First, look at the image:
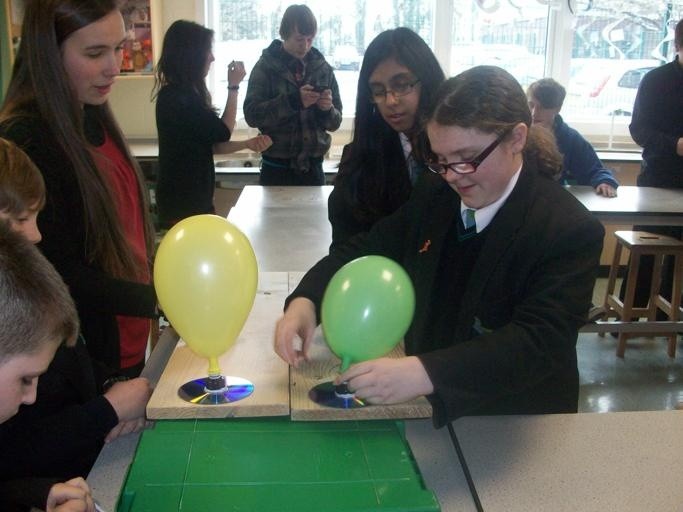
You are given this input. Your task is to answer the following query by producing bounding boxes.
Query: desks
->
[124,139,644,189]
[445,326,683,511]
[235,184,683,229]
[85,206,480,512]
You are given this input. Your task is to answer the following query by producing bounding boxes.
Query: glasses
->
[422,129,510,175]
[366,78,421,104]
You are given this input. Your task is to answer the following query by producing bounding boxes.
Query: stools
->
[597,230,683,358]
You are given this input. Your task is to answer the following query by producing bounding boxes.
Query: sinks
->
[593,150,642,155]
[213,156,262,175]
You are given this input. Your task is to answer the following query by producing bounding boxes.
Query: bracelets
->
[226,85,240,89]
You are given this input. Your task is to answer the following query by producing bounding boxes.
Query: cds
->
[178,375,253,405]
[308,381,368,409]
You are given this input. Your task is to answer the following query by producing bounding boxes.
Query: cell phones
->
[315,84,329,93]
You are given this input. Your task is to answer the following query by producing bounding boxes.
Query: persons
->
[1,220,96,512]
[273,64,608,412]
[2,4,164,384]
[526,77,618,325]
[0,134,155,511]
[149,20,272,230]
[243,3,343,186]
[329,27,458,256]
[619,19,683,333]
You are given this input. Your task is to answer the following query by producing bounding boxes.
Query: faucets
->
[609,112,618,150]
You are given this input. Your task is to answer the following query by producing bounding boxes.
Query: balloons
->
[153,214,259,378]
[320,255,417,372]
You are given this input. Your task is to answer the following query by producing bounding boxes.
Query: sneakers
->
[588,300,607,322]
